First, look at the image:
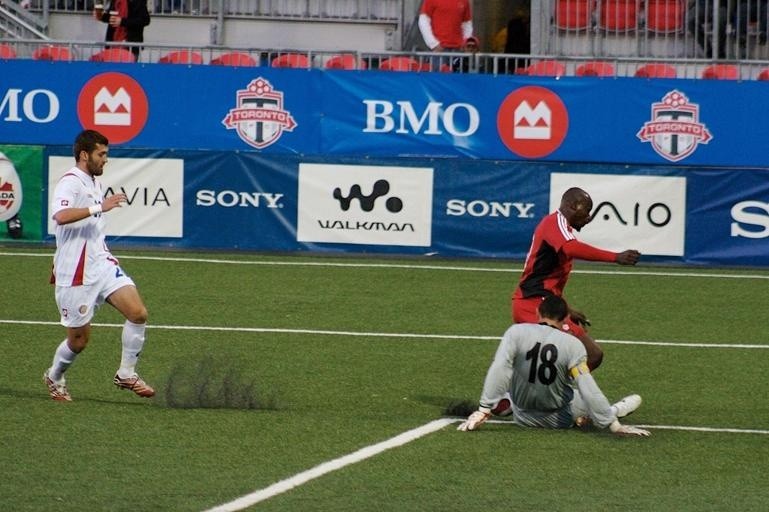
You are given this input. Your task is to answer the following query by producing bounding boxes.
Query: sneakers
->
[612,394,642,418]
[43,368,73,401]
[113,370,155,398]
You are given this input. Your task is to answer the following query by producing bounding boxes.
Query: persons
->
[456,294,650,437]
[418,0,484,73]
[94,0,149,56]
[490,188,641,417]
[42,129,156,403]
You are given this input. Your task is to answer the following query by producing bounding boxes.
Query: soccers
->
[490,388,514,416]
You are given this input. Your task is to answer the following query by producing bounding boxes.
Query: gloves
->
[456,405,491,432]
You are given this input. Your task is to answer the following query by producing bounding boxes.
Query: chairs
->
[0,0,769,80]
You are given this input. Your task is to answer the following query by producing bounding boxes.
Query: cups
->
[108,11,116,17]
[93,6,105,18]
